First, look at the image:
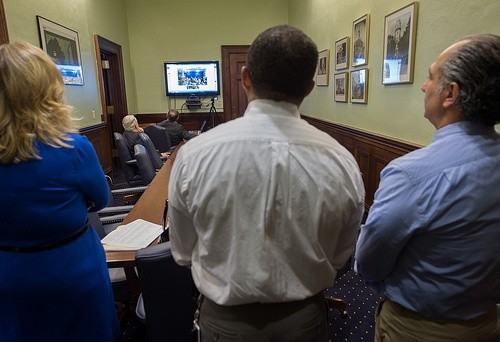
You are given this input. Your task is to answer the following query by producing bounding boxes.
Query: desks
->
[99,131,208,266]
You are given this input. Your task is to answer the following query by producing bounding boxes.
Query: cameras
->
[211,98,218,101]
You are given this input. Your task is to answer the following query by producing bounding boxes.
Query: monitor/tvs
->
[164,61,220,96]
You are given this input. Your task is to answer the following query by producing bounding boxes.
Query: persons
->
[167,25,366,342]
[122,114,169,174]
[354,30,364,59]
[354,33,500,342]
[0,40,121,342]
[158,109,201,147]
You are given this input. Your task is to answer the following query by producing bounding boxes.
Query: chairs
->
[104,172,148,216]
[87,211,118,241]
[139,131,165,172]
[144,124,176,154]
[133,144,158,184]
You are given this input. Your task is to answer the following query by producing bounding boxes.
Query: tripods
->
[200,101,220,132]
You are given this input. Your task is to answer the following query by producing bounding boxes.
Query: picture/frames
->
[349,67,369,104]
[381,1,420,86]
[333,71,348,103]
[316,49,331,87]
[35,15,85,87]
[350,12,370,67]
[334,36,350,70]
